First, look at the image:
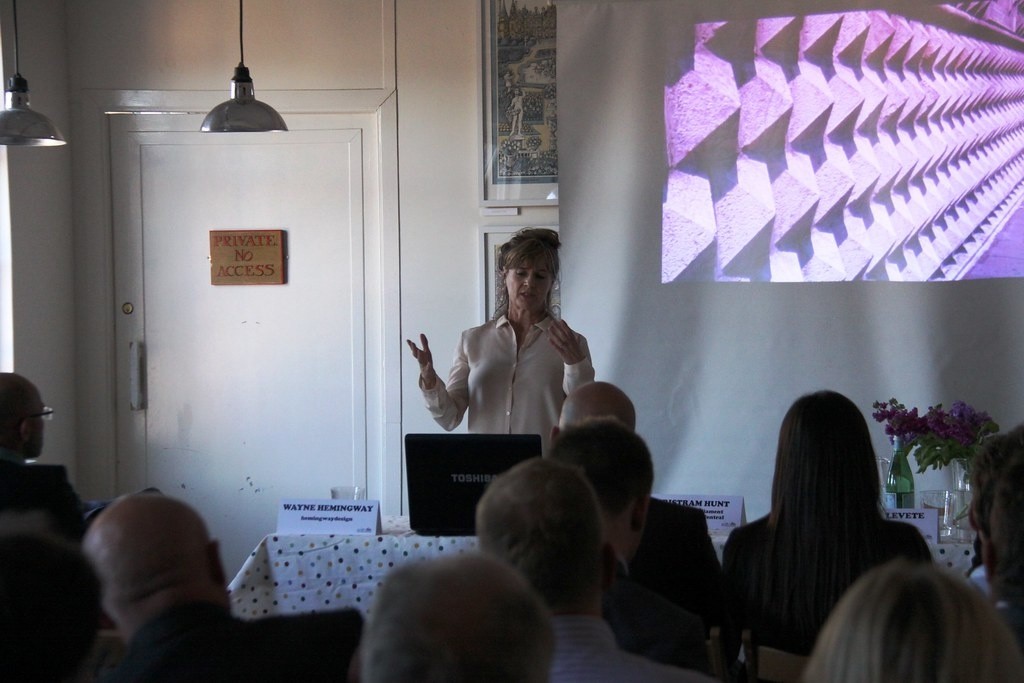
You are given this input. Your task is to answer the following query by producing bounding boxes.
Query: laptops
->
[404,433,542,537]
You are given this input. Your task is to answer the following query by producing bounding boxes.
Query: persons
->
[724,392,936,683]
[798,556,1024,683]
[468,455,723,683]
[0,512,107,683]
[405,227,596,468]
[351,549,556,683]
[546,381,728,643]
[83,490,365,682]
[963,424,1024,625]
[0,373,167,545]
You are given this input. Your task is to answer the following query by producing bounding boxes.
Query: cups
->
[875,457,888,496]
[921,491,977,544]
[331,487,365,501]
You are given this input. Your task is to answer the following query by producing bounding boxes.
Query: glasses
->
[14,406,52,428]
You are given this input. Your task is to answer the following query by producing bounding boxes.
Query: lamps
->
[1,0,67,145]
[198,0,289,132]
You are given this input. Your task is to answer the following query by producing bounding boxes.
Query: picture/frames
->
[941,458,977,543]
[473,0,559,206]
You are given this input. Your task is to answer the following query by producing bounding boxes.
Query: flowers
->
[872,398,942,455]
[914,401,1000,474]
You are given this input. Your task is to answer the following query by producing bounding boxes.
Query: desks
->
[228,514,976,620]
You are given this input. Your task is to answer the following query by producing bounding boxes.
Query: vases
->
[886,436,914,508]
